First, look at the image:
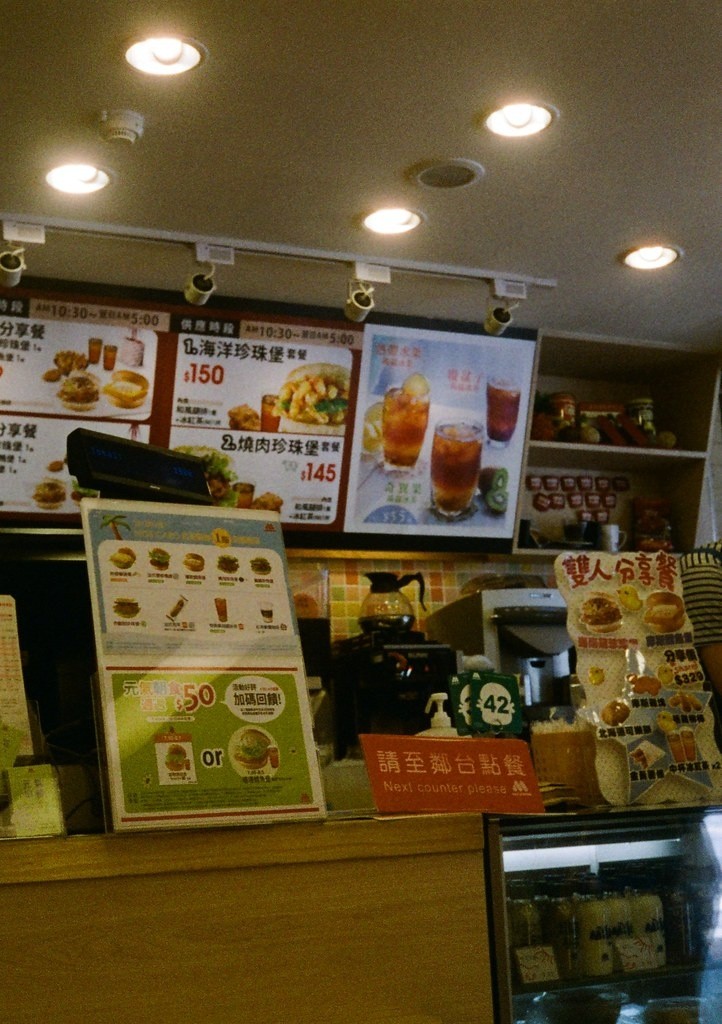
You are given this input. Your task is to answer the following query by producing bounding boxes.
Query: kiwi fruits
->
[478,466,510,514]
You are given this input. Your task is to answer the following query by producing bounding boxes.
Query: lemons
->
[402,372,430,395]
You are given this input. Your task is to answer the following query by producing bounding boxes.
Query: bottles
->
[507,881,702,994]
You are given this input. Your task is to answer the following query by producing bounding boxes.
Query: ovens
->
[422,585,585,729]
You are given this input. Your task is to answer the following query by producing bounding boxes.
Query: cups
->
[681,731,697,762]
[214,598,228,622]
[485,378,522,449]
[261,393,281,434]
[102,344,117,370]
[88,339,103,363]
[601,523,628,554]
[185,758,191,771]
[268,747,279,768]
[259,601,274,623]
[668,733,686,763]
[430,417,484,518]
[383,386,431,472]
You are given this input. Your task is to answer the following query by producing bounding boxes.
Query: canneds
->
[544,391,656,438]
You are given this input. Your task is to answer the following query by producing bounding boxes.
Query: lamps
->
[0,241,28,288]
[484,298,520,337]
[344,279,375,323]
[183,263,216,307]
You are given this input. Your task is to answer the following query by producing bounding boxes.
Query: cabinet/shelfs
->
[511,326,722,556]
[486,804,722,1024]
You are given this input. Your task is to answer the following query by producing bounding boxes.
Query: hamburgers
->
[102,370,149,409]
[270,362,352,436]
[31,478,67,510]
[234,729,271,770]
[578,592,686,634]
[147,548,272,576]
[164,743,187,772]
[112,598,141,618]
[70,480,99,507]
[55,370,100,410]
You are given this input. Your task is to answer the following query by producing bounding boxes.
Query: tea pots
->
[358,570,427,636]
